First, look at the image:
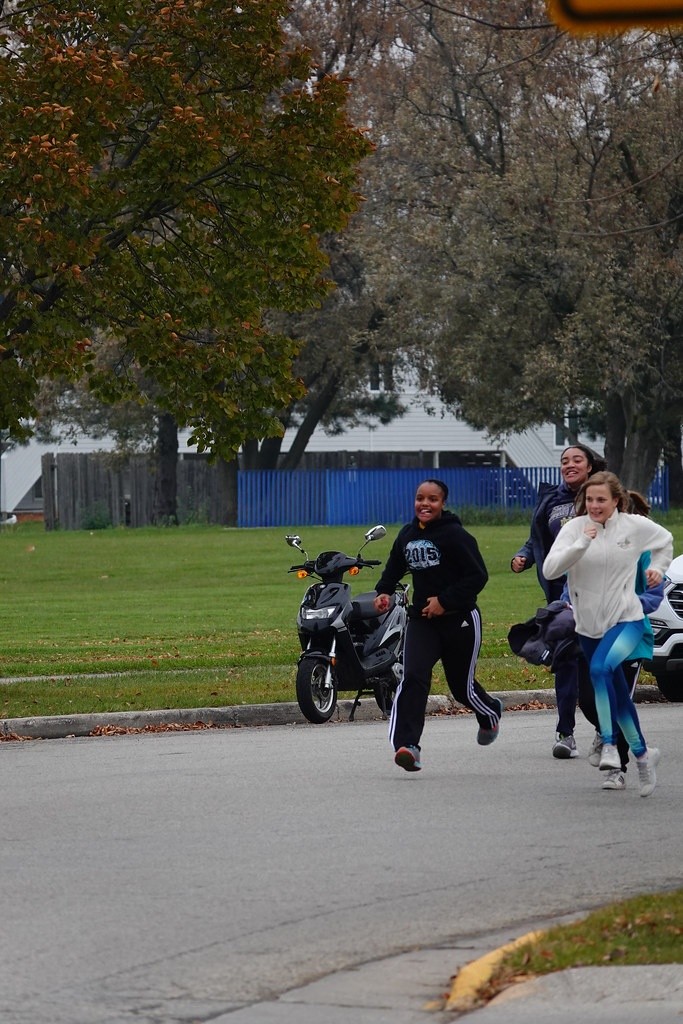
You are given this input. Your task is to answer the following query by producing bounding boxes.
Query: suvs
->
[638,555,683,703]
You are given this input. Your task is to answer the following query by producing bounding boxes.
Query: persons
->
[375,479,502,771]
[511,446,607,758]
[542,470,674,796]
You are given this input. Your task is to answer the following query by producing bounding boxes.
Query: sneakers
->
[588,731,603,767]
[553,735,579,759]
[602,768,627,789]
[598,743,621,771]
[477,698,502,746]
[395,747,422,773]
[636,747,660,796]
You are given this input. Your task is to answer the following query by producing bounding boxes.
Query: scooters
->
[284,524,411,725]
